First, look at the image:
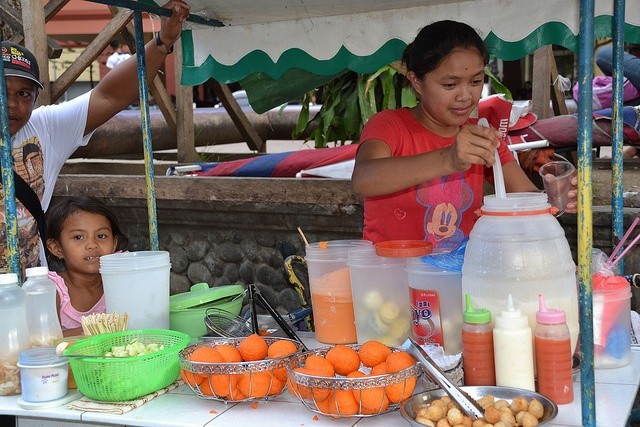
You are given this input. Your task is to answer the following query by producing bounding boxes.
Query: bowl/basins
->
[170,287,246,338]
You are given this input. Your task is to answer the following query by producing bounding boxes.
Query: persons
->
[45,194,129,337]
[0,1,191,276]
[106,39,134,109]
[350,19,578,252]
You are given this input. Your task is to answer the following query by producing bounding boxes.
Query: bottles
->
[461,292,495,387]
[0,273,29,397]
[534,293,574,405]
[492,293,535,392]
[25,265,63,356]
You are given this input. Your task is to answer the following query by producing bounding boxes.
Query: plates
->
[398,385,559,427]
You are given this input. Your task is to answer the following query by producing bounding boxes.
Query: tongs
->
[247,282,310,353]
[398,336,491,423]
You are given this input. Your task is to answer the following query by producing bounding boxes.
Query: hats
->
[0,40,45,91]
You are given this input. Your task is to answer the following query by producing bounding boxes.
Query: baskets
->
[63,329,191,401]
[286,341,422,418]
[178,335,304,403]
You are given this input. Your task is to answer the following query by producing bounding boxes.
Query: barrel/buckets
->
[462,190,579,360]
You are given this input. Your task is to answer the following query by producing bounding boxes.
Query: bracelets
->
[155,31,174,55]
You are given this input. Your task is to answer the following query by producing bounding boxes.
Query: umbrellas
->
[295,91,550,180]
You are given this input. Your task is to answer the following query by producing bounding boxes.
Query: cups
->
[587,269,634,370]
[15,344,71,404]
[538,160,575,211]
